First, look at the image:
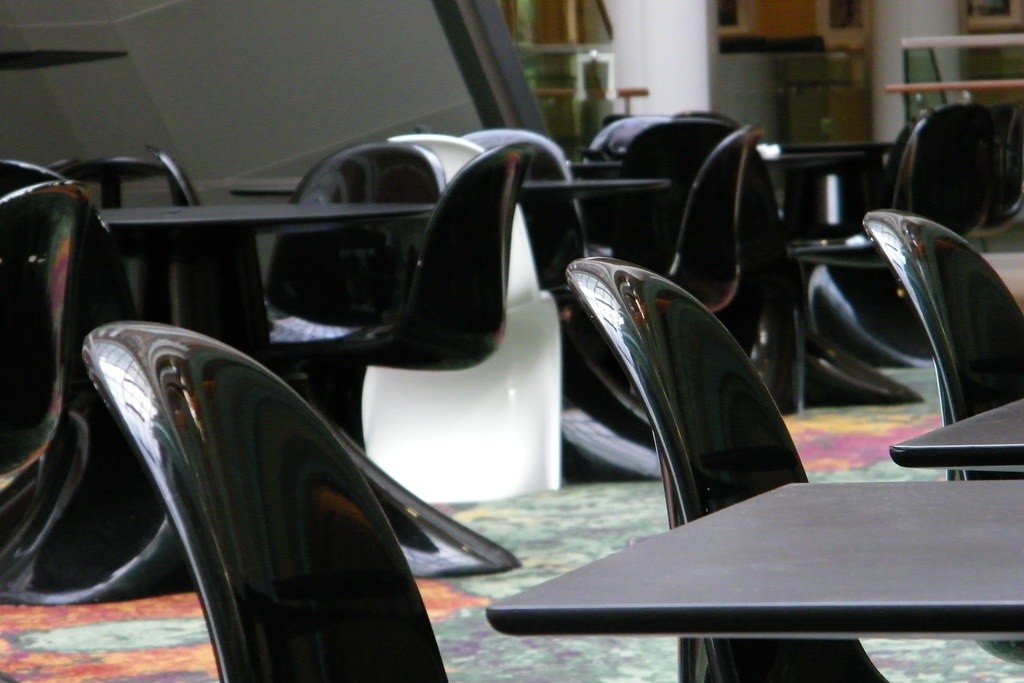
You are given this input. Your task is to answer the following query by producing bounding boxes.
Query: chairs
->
[0,102,1024,683]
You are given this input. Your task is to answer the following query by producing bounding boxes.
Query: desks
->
[890,398,1024,471]
[67,203,521,606]
[566,152,923,407]
[486,482,1024,683]
[228,179,667,477]
[757,142,929,367]
